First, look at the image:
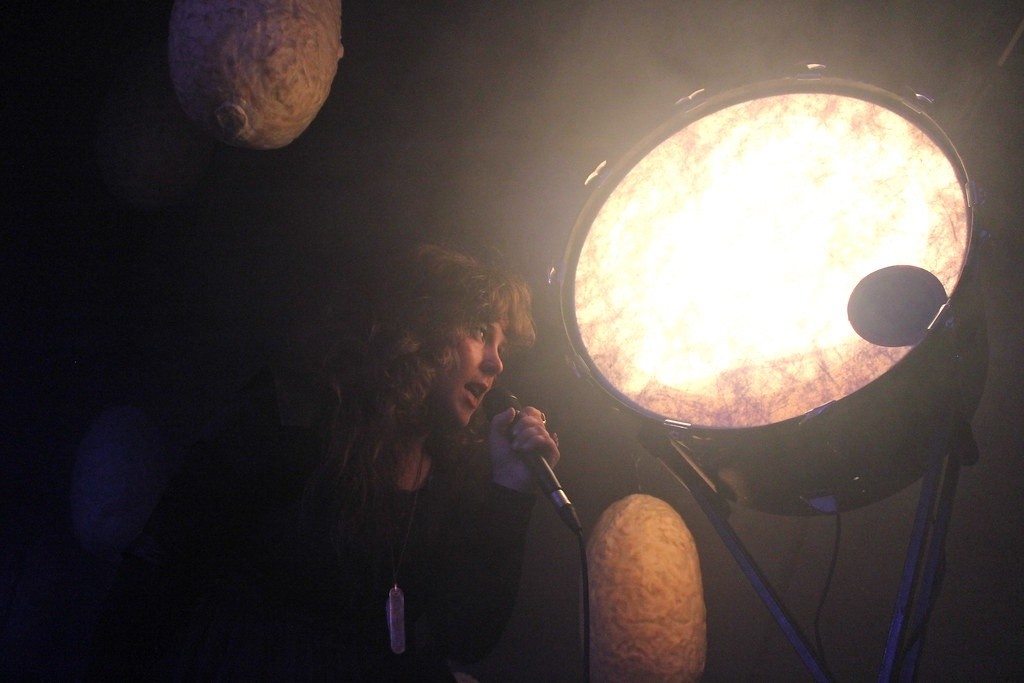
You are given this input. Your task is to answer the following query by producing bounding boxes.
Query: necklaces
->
[362,439,429,656]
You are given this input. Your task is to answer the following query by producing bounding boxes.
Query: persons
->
[83,242,562,683]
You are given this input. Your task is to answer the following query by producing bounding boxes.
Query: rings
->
[541,412,547,426]
[552,432,559,447]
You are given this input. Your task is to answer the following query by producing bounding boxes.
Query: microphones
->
[483,385,581,534]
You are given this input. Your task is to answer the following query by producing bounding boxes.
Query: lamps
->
[549,62,985,683]
